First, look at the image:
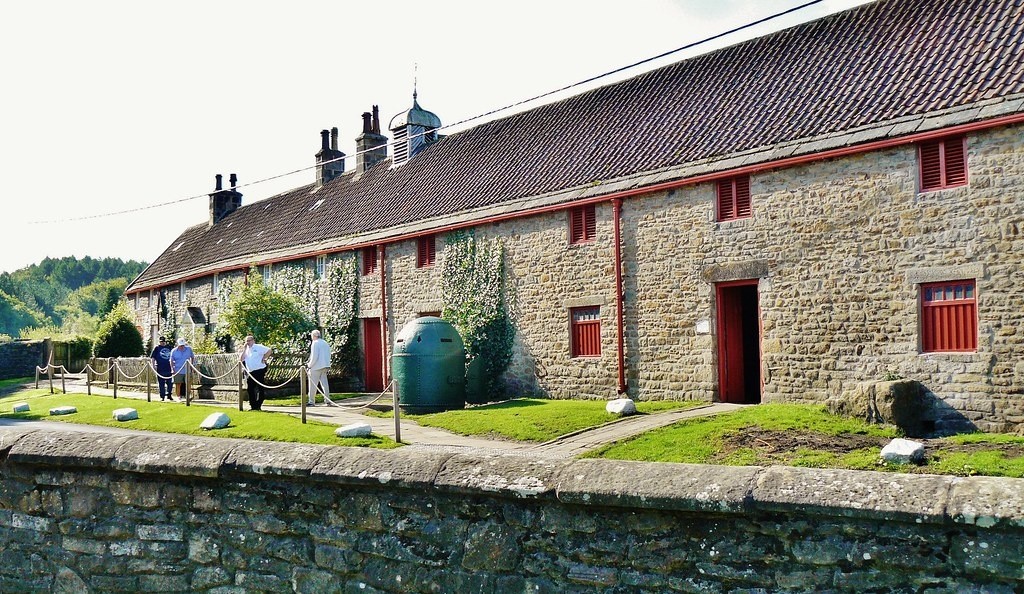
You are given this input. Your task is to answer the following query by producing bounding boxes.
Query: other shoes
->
[167,394,174,400]
[323,401,331,407]
[161,397,165,401]
[306,402,315,407]
[247,406,261,411]
[175,395,182,403]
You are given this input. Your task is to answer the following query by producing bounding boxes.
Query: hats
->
[177,338,185,347]
[158,336,165,342]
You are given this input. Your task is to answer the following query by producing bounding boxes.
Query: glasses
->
[182,345,184,346]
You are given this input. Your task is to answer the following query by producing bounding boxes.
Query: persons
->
[150,336,173,401]
[240,336,272,411]
[170,338,194,403]
[307,329,331,407]
